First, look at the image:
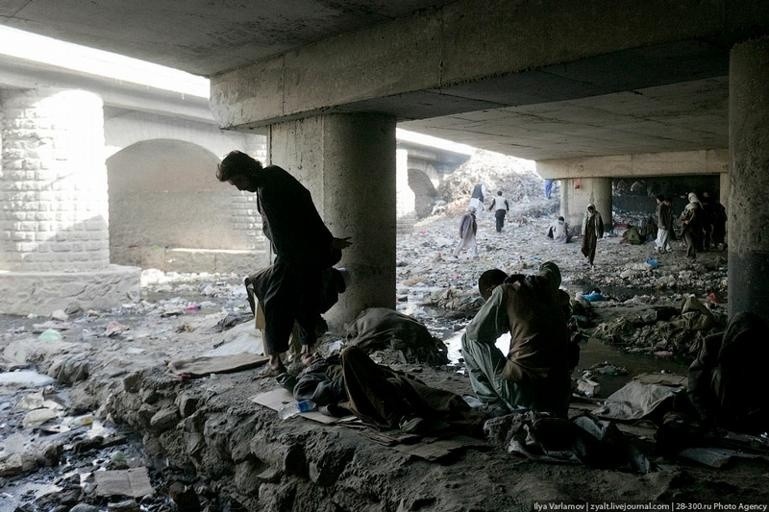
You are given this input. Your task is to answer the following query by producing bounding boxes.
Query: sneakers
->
[398,414,426,433]
[250,362,288,382]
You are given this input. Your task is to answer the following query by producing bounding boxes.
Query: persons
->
[487,191,509,232]
[685,304,769,439]
[215,151,355,381]
[547,216,572,243]
[581,203,604,266]
[453,205,479,260]
[287,345,532,442]
[459,261,582,418]
[653,191,727,262]
[244,265,352,365]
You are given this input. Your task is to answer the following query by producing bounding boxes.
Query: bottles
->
[278,398,317,421]
[185,305,201,310]
